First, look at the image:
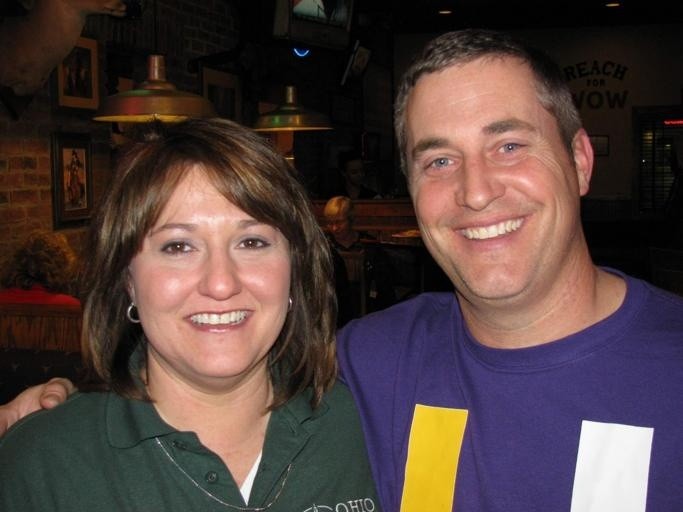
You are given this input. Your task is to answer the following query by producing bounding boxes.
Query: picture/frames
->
[197,56,246,128]
[51,30,106,231]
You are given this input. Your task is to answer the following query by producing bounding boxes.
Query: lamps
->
[92,1,220,121]
[251,0,335,132]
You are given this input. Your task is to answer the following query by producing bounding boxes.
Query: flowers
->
[4,228,78,294]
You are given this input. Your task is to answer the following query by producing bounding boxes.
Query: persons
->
[0,28,683,512]
[320,150,408,328]
[0,231,82,308]
[0,118,382,511]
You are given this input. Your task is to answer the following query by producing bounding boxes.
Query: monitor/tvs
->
[271,0,356,50]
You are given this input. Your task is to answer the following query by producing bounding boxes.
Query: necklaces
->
[155,433,292,511]
